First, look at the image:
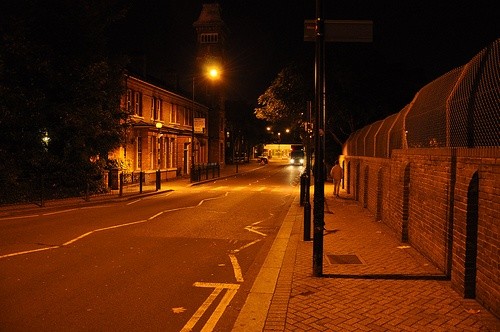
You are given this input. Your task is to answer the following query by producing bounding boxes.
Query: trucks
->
[290,145,305,167]
[234,151,251,163]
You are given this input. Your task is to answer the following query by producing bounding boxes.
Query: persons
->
[330,160,343,198]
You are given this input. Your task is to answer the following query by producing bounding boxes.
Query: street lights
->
[191,62,220,181]
